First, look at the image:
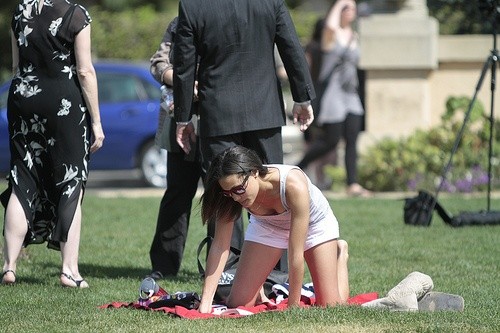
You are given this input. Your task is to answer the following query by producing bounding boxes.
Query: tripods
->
[418,8,500,229]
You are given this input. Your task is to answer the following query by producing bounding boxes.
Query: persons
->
[174,0,315,282]
[144,16,200,281]
[193,146,350,315]
[296,0,373,198]
[0,0,105,288]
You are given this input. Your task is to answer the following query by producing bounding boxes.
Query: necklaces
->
[250,183,266,212]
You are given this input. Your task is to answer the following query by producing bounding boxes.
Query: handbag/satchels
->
[304,79,329,120]
[197,236,289,306]
[139,292,200,310]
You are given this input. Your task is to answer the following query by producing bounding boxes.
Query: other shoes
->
[352,187,373,198]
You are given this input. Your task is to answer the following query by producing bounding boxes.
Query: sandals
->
[60,272,89,289]
[2,270,16,286]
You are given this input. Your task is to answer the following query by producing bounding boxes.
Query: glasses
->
[222,171,250,197]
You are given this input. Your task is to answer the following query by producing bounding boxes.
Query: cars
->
[0,63,168,189]
